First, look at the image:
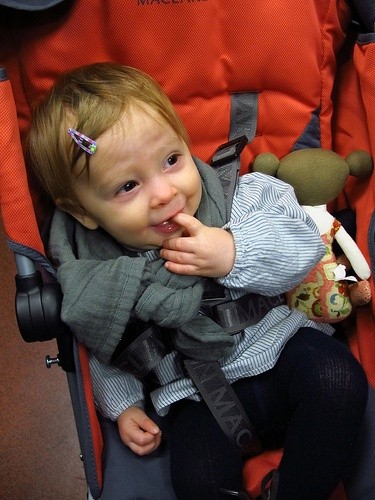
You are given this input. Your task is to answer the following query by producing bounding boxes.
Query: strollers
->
[1,0,375,500]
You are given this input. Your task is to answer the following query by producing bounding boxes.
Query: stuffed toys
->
[252,148,373,323]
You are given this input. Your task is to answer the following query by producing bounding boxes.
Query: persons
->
[26,60,374,500]
